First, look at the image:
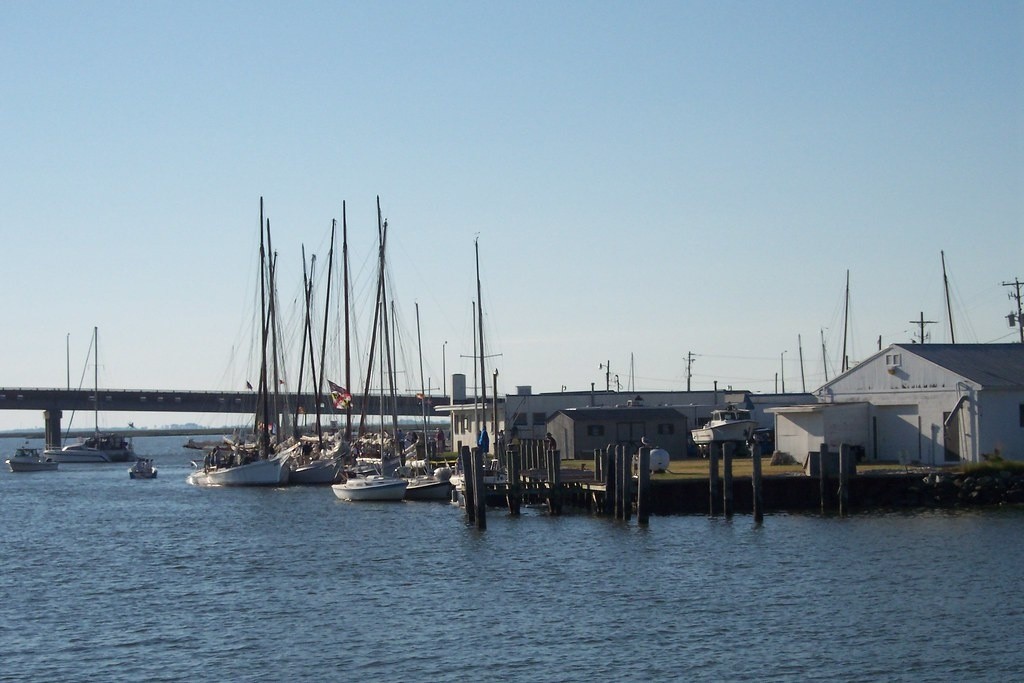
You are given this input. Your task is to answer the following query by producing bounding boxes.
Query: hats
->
[546,433,553,436]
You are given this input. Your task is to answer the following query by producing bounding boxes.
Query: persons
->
[303,441,312,467]
[398,426,445,456]
[136,457,153,471]
[498,430,506,456]
[204,446,221,475]
[546,432,556,450]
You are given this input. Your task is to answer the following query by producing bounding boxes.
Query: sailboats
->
[40,325,139,463]
[182,194,490,504]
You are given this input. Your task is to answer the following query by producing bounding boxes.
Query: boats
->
[128,457,159,480]
[690,401,758,441]
[64,331,72,391]
[8,445,59,473]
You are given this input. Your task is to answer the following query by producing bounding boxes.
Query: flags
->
[247,382,252,390]
[327,379,353,410]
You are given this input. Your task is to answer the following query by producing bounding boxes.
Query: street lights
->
[599,359,613,391]
[441,340,449,396]
